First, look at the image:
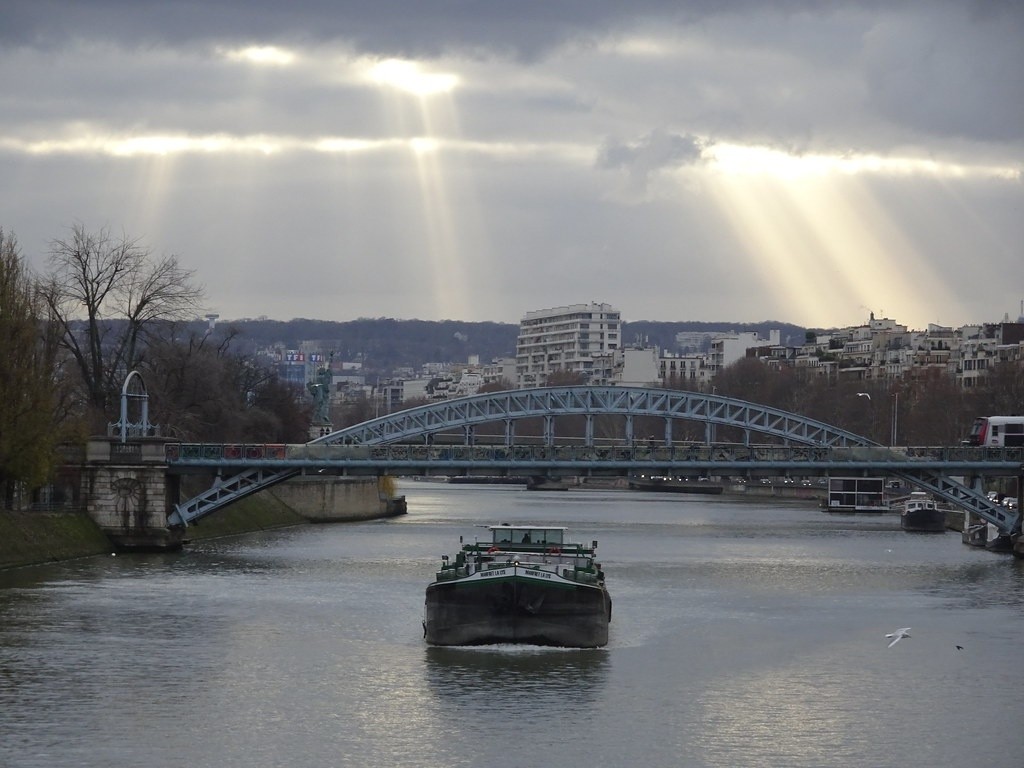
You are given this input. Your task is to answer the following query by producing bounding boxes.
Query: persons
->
[522,533,530,544]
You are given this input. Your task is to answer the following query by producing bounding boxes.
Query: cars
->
[1001,496,1013,508]
[1007,499,1018,510]
[988,492,1000,504]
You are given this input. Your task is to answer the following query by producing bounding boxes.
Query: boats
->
[421,520,613,649]
[900,491,950,532]
[628,474,724,494]
[526,475,569,491]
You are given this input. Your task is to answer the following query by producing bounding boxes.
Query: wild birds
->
[956,645,964,652]
[884,627,913,648]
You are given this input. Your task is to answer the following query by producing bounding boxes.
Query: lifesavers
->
[549,547,561,557]
[488,546,500,556]
